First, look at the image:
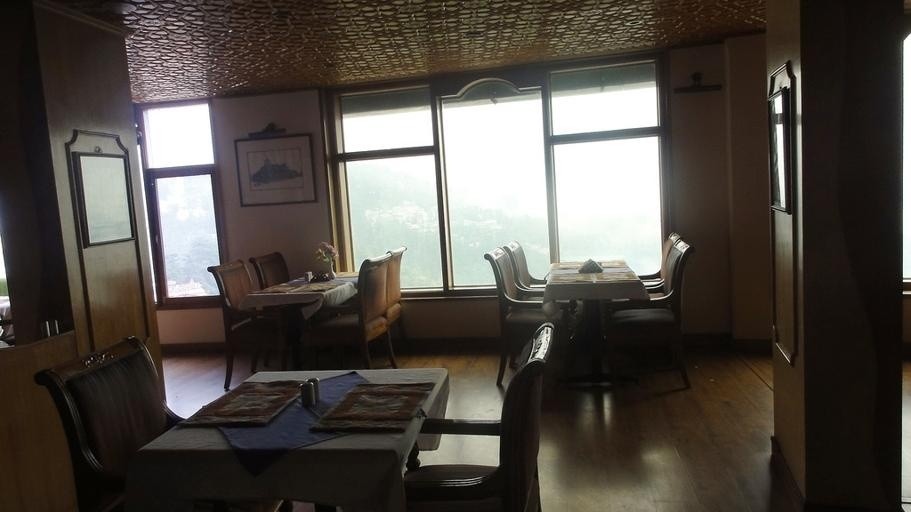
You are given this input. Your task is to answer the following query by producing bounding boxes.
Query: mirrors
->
[73,151,136,247]
[768,86,793,215]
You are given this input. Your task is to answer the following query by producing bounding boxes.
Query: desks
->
[135,367,455,510]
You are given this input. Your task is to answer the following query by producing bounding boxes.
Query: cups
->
[300,377,320,407]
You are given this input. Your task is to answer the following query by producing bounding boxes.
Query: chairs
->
[480,231,695,392]
[206,241,409,392]
[310,320,557,512]
[32,331,294,511]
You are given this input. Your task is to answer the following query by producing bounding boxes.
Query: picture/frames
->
[233,132,317,210]
